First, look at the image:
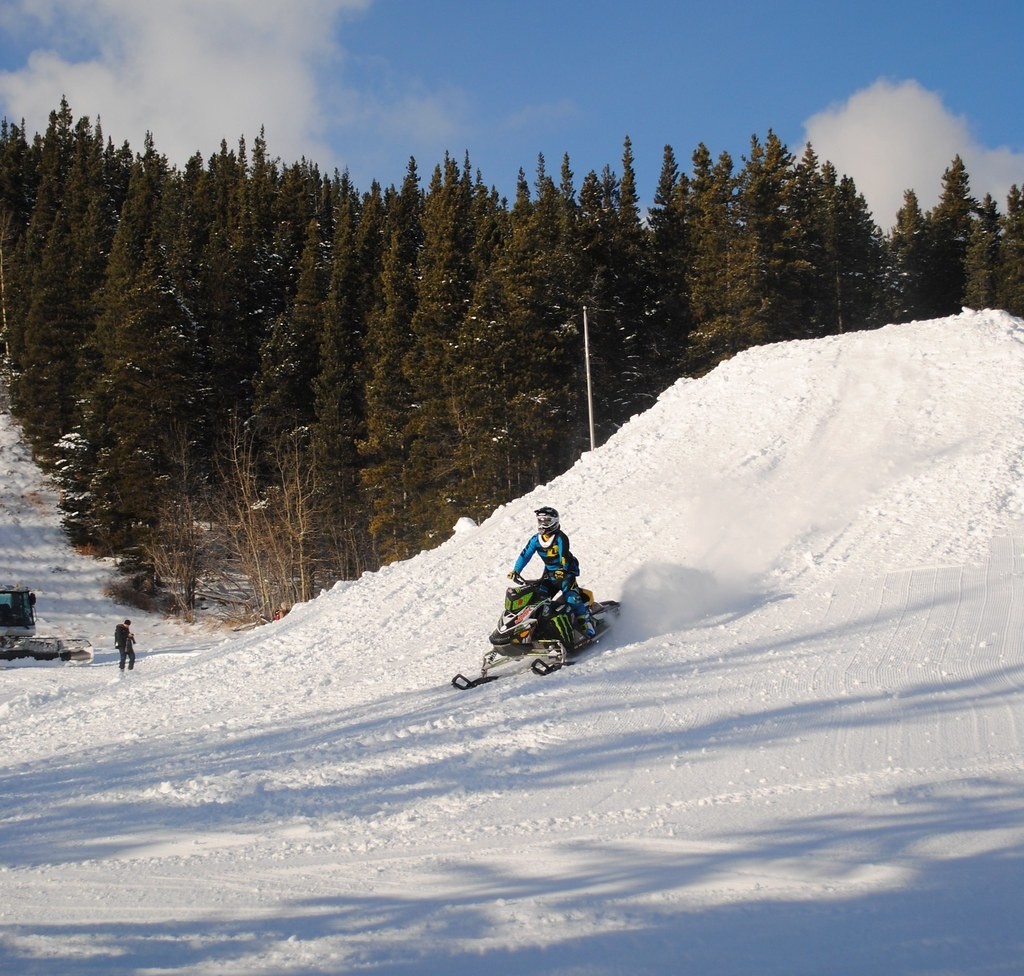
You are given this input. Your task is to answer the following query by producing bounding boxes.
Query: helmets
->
[534,506,559,536]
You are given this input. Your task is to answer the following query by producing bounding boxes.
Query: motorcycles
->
[451,571,622,691]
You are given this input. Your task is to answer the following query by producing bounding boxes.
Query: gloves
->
[555,569,564,579]
[507,571,520,579]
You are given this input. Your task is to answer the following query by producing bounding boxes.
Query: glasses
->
[537,516,556,528]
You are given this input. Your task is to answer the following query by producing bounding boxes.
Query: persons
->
[115,620,136,671]
[507,507,595,638]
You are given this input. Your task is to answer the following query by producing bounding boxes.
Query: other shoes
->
[585,614,595,637]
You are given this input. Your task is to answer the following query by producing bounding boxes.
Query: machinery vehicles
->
[0,585,95,662]
